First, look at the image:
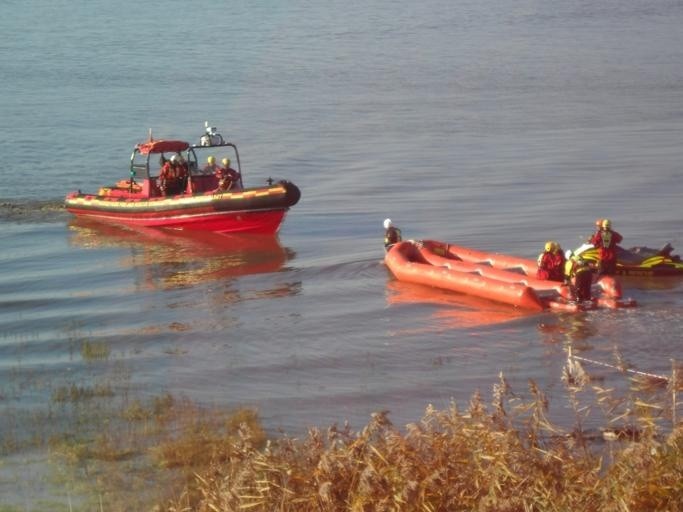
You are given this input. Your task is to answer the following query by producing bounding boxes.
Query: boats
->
[564,241,682,277]
[67,218,288,292]
[63,120,302,235]
[385,279,535,337]
[588,272,682,291]
[385,238,636,315]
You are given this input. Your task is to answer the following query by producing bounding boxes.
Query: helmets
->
[564,249,575,261]
[169,154,180,163]
[544,239,561,256]
[207,155,217,164]
[595,217,612,228]
[383,218,394,230]
[221,157,231,166]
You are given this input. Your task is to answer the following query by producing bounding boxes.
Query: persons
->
[156,155,242,194]
[536,218,623,305]
[381,218,402,252]
